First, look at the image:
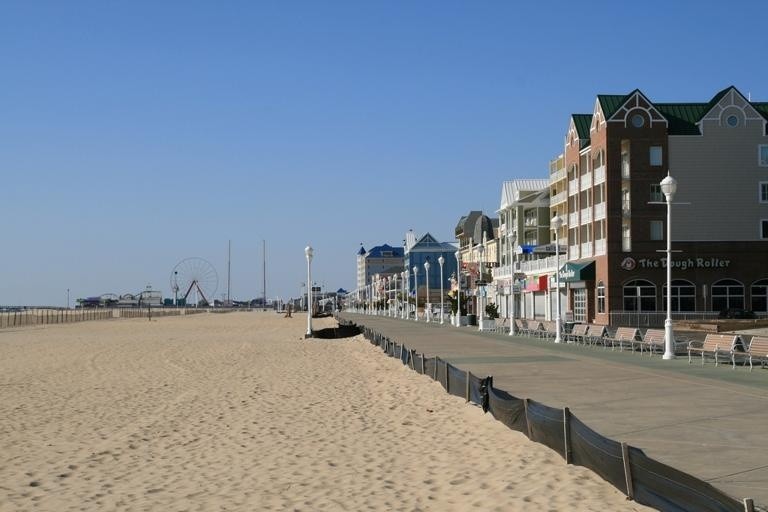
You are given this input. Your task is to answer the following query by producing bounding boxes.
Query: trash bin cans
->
[467,314,477,325]
[566,322,581,342]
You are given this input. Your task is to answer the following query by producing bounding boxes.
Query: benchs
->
[686,333,768,370]
[565,323,690,360]
[477,318,564,342]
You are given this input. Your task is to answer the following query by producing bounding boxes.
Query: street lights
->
[475,242,488,332]
[343,269,411,320]
[423,259,432,323]
[659,167,677,361]
[412,264,420,321]
[454,248,464,327]
[304,242,315,339]
[550,207,566,345]
[506,228,517,337]
[438,253,446,326]
